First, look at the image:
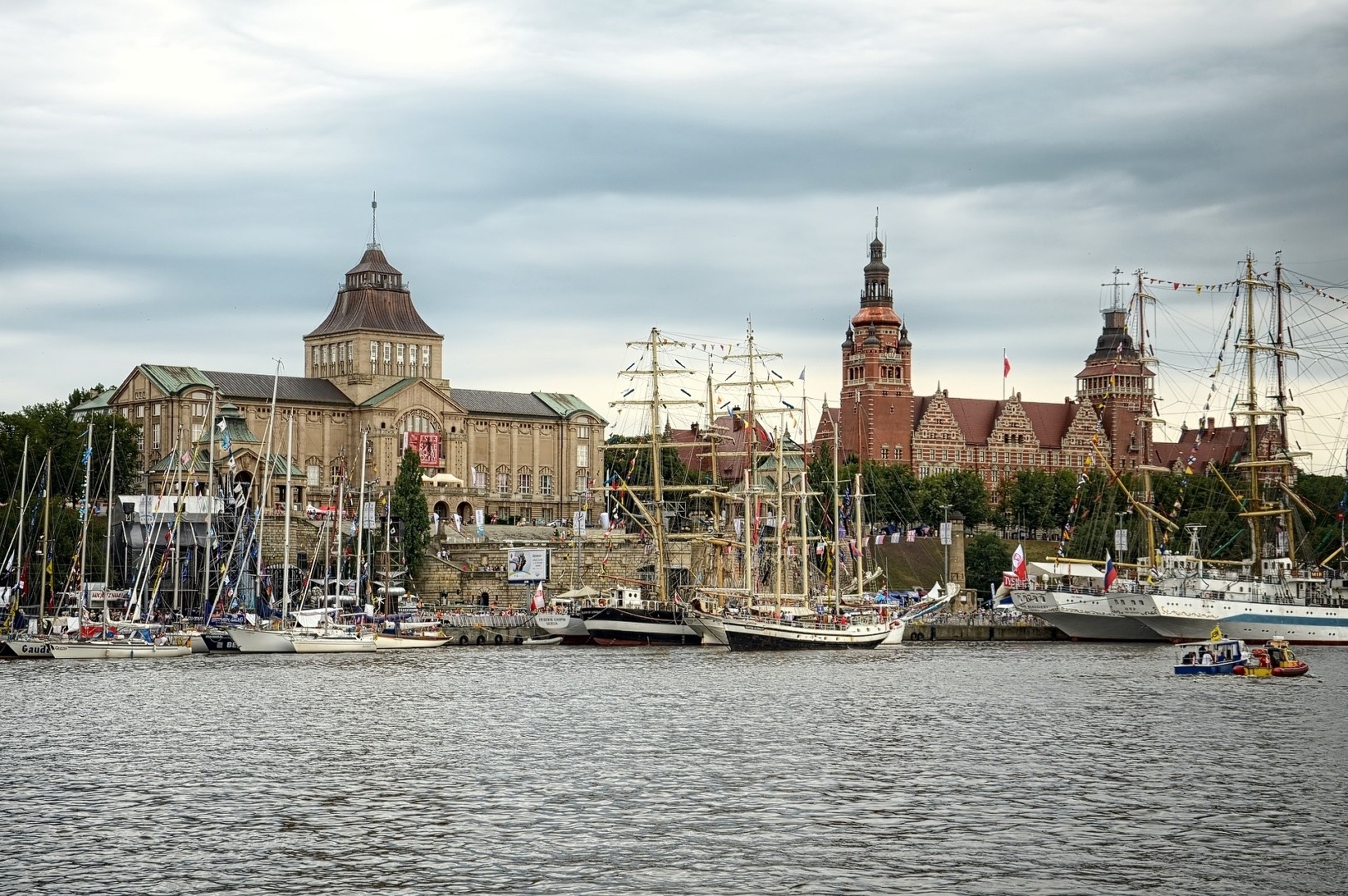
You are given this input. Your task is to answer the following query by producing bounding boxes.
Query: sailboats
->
[0,249,1348,660]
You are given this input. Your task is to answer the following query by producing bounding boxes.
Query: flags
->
[1004,349,1011,378]
[1012,544,1029,581]
[1104,551,1118,592]
[531,582,546,612]
[181,420,275,610]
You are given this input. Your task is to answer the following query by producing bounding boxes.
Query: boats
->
[1232,636,1308,677]
[1172,625,1251,676]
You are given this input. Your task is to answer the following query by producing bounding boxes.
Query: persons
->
[432,513,572,540]
[436,547,508,571]
[91,610,177,623]
[436,606,569,615]
[723,604,1044,624]
[306,510,350,521]
[1183,645,1272,667]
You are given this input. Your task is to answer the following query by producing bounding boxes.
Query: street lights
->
[938,504,955,584]
[571,490,588,591]
